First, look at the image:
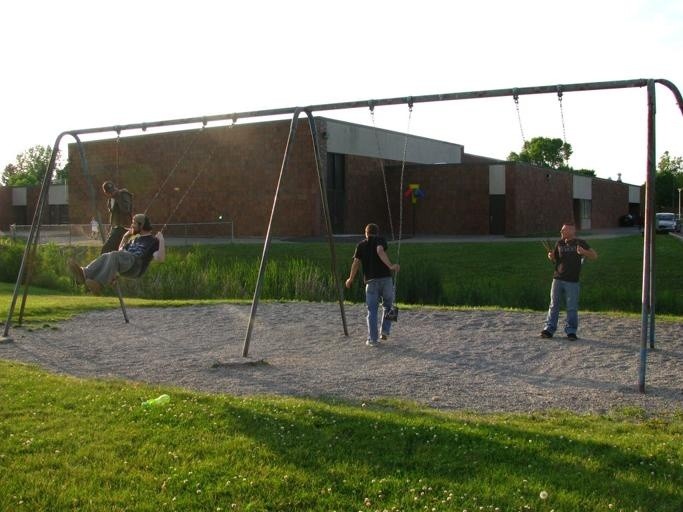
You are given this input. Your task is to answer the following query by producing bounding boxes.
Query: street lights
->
[677,188,682,221]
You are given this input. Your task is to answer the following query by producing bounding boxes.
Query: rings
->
[577,252,580,254]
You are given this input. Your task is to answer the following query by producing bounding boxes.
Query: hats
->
[103,182,114,193]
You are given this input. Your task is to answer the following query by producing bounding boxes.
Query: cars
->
[655,212,677,233]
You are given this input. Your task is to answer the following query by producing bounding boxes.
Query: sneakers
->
[69,262,85,284]
[366,335,387,347]
[85,279,101,291]
[541,330,551,338]
[569,333,577,341]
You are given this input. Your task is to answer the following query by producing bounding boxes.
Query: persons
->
[345,224,400,347]
[89,217,98,240]
[100,181,131,256]
[539,222,596,340]
[71,214,165,294]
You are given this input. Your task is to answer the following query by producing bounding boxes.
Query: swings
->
[114,120,236,279]
[368,105,412,321]
[514,96,581,283]
